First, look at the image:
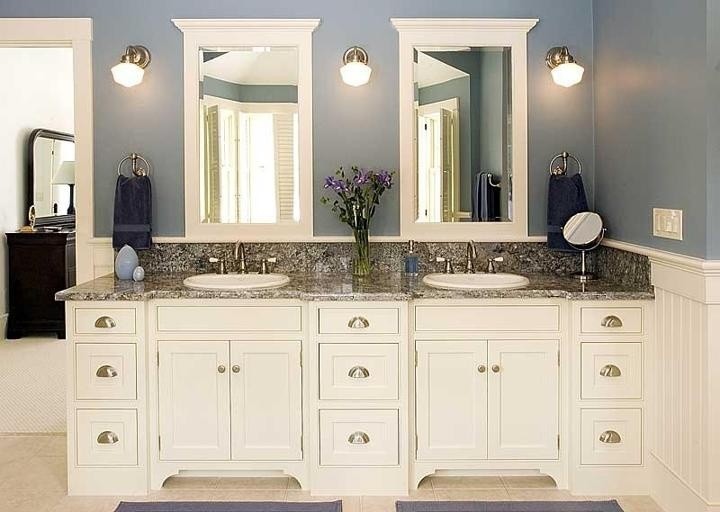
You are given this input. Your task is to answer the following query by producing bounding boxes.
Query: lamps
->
[546,46,585,89]
[50,161,76,214]
[111,45,150,87]
[339,46,373,87]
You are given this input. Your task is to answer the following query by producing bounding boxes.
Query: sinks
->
[423,271,530,290]
[183,271,289,290]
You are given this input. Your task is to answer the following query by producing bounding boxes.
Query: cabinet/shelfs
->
[408,303,572,492]
[149,297,308,490]
[64,298,149,496]
[4,227,76,341]
[573,299,652,497]
[309,301,409,496]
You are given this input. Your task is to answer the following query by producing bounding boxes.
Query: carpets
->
[395,499,624,512]
[115,499,345,511]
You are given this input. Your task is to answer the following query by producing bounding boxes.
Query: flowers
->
[319,163,396,271]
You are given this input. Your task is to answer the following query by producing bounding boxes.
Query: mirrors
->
[561,212,608,280]
[28,129,76,229]
[390,16,539,237]
[170,17,321,238]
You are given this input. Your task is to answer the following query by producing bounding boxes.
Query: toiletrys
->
[405,240,418,276]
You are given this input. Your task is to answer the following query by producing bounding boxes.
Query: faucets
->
[235,241,248,274]
[464,240,478,273]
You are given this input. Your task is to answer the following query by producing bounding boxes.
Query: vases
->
[350,227,372,276]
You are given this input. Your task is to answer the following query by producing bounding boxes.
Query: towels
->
[472,173,497,220]
[546,173,592,252]
[111,174,155,251]
